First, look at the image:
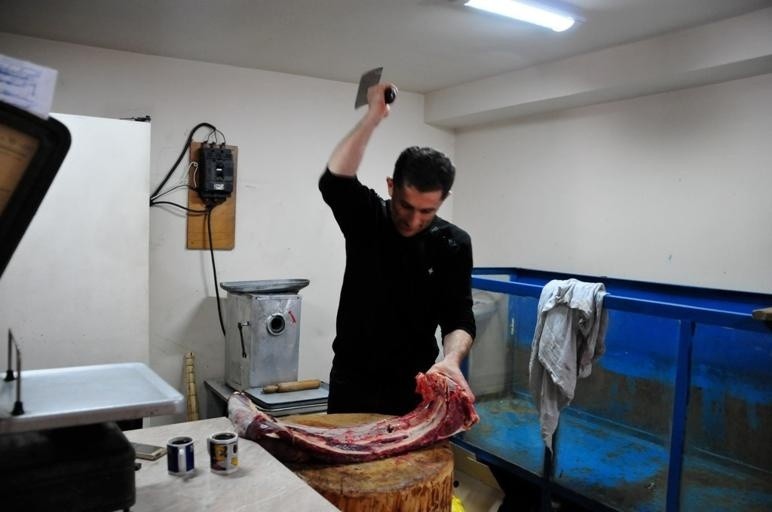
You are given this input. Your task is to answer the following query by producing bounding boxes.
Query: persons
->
[320,79,475,415]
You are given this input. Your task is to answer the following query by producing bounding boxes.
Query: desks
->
[119,416,344,512]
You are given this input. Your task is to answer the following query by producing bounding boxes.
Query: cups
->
[165,435,195,477]
[207,430,239,476]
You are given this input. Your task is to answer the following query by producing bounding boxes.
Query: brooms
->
[182,350,201,422]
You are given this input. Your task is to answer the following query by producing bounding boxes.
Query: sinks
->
[1,361,187,435]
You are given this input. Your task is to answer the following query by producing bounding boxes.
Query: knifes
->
[352,66,397,110]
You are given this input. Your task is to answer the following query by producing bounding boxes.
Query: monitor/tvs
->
[0,99,71,277]
